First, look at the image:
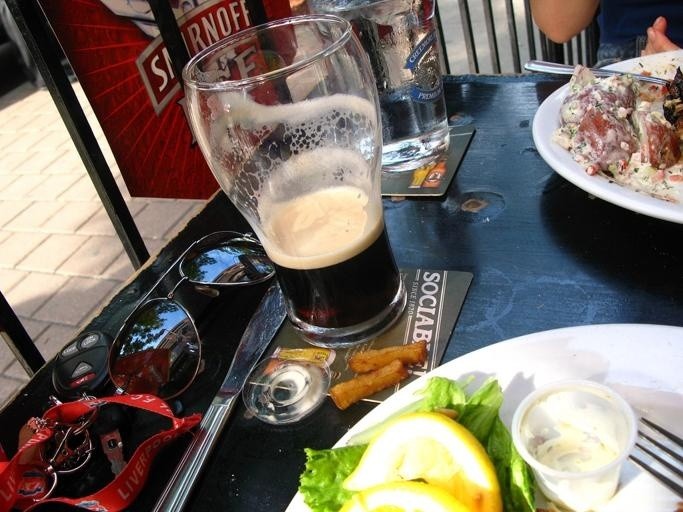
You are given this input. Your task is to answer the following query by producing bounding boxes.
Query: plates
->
[276,323,682,512]
[532,48,683,226]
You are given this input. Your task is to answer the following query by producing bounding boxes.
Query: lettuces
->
[298,375,536,512]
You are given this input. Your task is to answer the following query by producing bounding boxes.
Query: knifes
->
[152,286,286,512]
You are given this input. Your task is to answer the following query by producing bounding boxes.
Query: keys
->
[94,403,127,477]
[51,330,114,399]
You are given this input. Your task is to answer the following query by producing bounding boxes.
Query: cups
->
[306,0,453,173]
[513,379,637,512]
[181,11,410,352]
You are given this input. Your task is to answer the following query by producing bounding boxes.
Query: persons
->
[530,0,683,68]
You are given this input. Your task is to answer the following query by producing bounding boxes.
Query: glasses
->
[16,407,97,505]
[108,230,275,400]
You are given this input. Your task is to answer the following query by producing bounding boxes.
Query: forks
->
[629,417,683,496]
